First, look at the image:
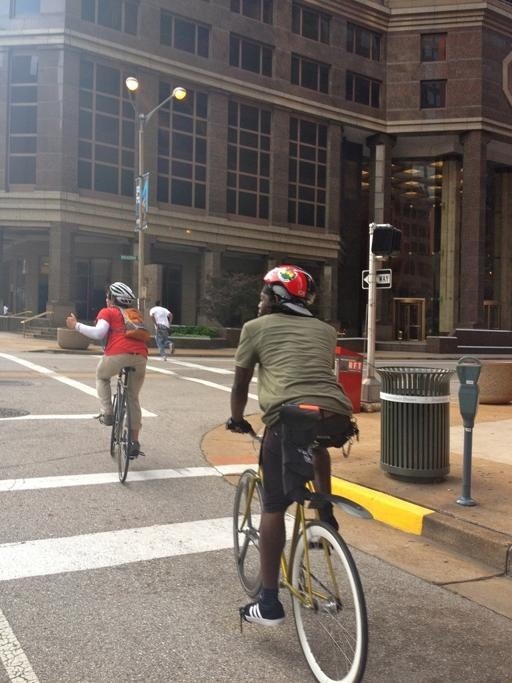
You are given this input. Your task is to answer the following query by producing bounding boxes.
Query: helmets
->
[110,282,137,305]
[263,264,316,316]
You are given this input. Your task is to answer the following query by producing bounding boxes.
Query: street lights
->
[124,75,187,312]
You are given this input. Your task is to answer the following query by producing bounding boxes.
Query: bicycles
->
[231,416,369,682]
[91,337,141,482]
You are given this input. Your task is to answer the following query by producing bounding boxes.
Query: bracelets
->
[228,417,246,428]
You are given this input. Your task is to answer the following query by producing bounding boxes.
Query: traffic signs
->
[362,268,393,289]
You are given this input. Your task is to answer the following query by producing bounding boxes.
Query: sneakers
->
[162,343,174,361]
[242,599,285,625]
[99,414,113,426]
[128,440,140,456]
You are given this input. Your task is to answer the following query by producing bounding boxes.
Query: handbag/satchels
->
[279,403,322,430]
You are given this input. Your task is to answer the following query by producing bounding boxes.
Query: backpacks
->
[116,306,152,341]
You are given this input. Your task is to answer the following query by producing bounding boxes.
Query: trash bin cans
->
[397,331,403,340]
[376,366,457,483]
[335,346,363,413]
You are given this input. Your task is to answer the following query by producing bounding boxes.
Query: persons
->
[64,279,150,460]
[227,263,357,627]
[3,302,11,316]
[148,299,175,362]
